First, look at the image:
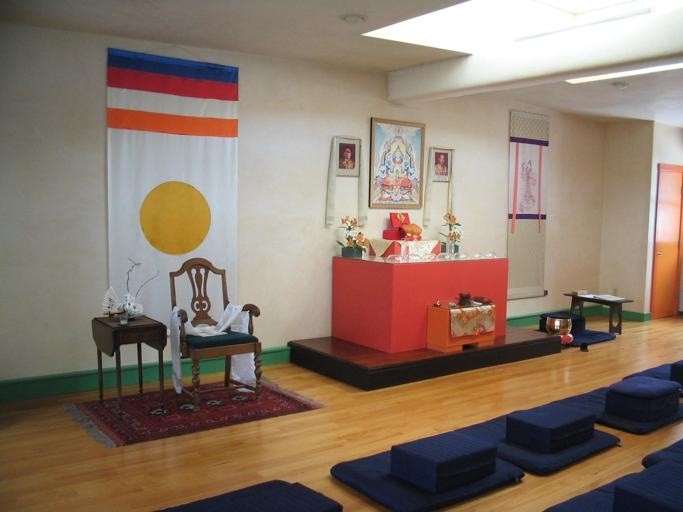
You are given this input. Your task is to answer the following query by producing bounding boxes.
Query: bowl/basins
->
[546,316,573,337]
[386,252,496,262]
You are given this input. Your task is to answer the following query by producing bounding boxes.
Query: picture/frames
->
[368,116,426,210]
[335,136,360,177]
[431,148,451,182]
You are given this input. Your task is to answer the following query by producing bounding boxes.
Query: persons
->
[339,147,355,169]
[435,154,448,176]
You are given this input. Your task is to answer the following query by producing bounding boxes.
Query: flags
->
[107,48,239,329]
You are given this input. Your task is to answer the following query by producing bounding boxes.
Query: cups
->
[119,310,128,325]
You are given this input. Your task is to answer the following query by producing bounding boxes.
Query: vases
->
[441,244,459,254]
[342,247,362,259]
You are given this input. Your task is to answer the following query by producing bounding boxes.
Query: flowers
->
[335,215,370,253]
[439,212,464,243]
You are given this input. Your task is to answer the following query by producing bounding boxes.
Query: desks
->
[427,303,496,353]
[91,316,168,423]
[563,293,634,335]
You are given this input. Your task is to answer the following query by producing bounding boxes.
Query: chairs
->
[168,256,262,405]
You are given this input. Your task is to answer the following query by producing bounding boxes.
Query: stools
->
[396,429,498,492]
[203,481,344,512]
[669,360,682,376]
[603,375,682,420]
[613,457,682,511]
[539,311,586,336]
[505,402,594,453]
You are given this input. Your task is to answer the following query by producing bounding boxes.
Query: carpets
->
[63,375,329,449]
